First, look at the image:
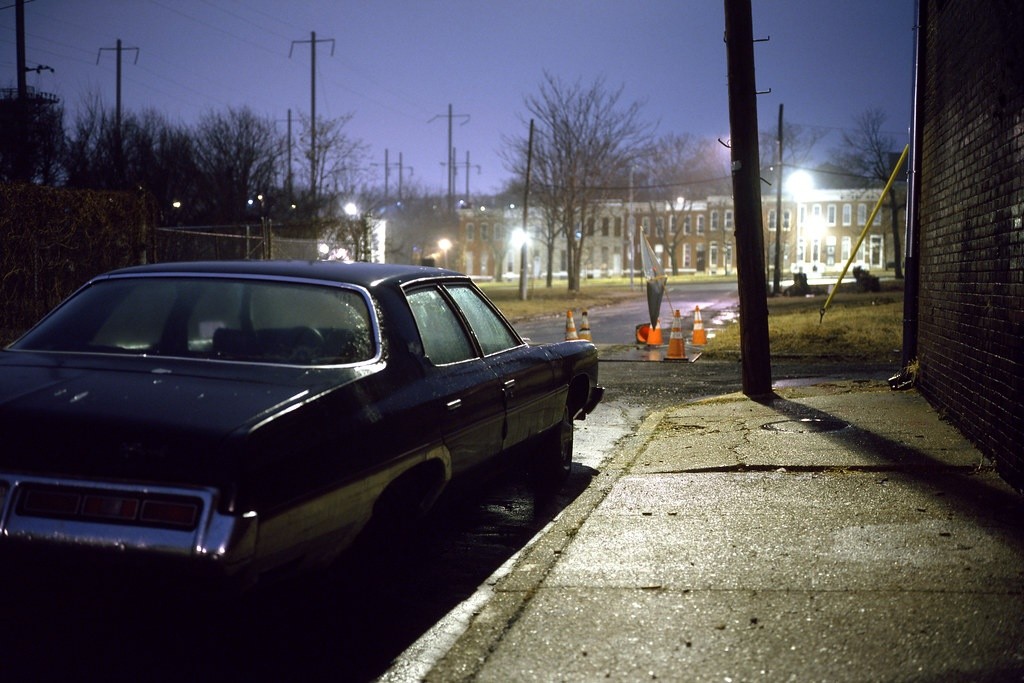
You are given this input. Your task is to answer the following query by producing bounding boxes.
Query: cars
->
[0,259,607,618]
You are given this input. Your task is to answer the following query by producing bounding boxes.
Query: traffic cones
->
[692,305,708,345]
[636,311,664,345]
[663,310,690,360]
[566,308,578,340]
[579,306,593,343]
